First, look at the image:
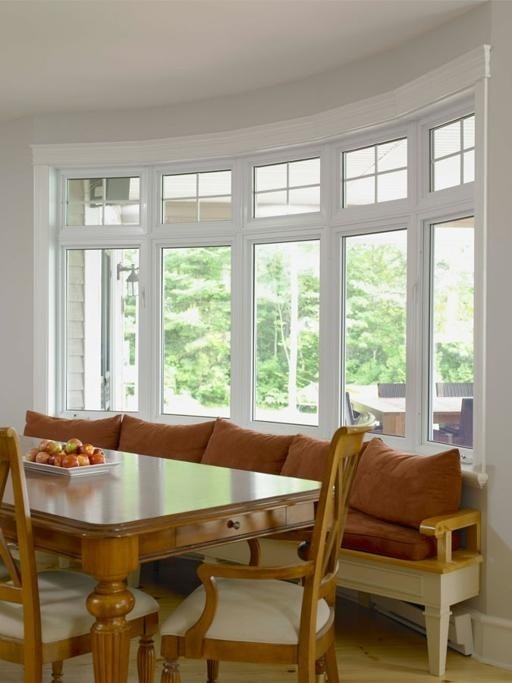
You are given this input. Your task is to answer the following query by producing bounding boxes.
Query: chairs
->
[344,381,473,447]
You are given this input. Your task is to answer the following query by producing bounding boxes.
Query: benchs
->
[22,411,483,677]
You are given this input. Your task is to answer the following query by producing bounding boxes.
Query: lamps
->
[115,263,140,295]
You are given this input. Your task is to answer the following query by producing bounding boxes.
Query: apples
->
[25,437,105,468]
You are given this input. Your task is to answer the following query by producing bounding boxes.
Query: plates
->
[22,455,121,478]
